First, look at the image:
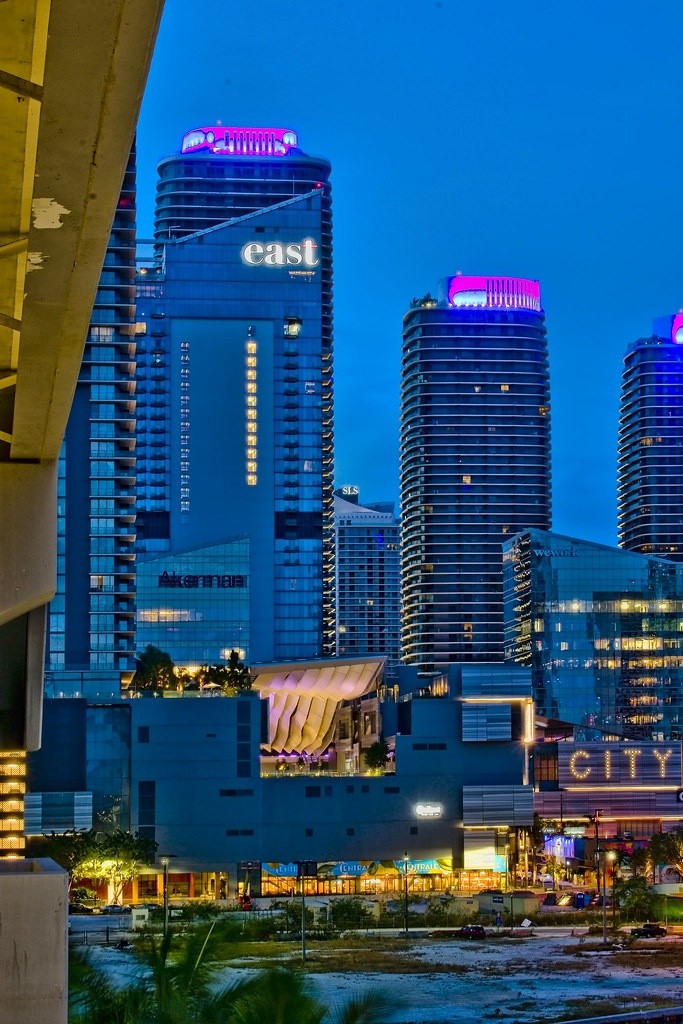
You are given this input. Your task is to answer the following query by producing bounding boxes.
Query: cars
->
[102,904,130,915]
[146,903,158,912]
[68,903,93,915]
[457,925,486,940]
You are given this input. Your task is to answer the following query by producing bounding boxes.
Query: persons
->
[242,892,250,905]
[220,880,227,899]
[275,756,327,773]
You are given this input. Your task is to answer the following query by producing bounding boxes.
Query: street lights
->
[608,851,617,928]
[403,850,410,932]
[160,857,169,937]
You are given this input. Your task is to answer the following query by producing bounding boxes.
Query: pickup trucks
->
[630,924,667,938]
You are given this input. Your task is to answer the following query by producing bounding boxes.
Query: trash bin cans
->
[574,892,584,908]
[547,893,556,905]
[584,895,590,905]
